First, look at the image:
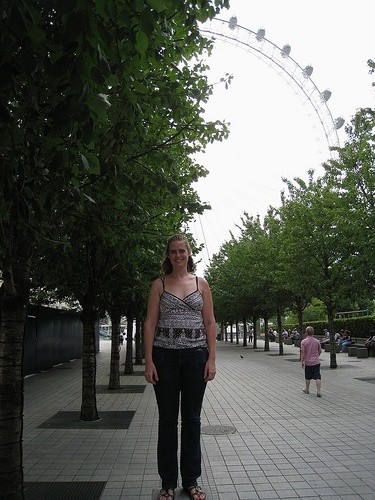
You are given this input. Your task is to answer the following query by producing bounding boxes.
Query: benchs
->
[311,335,369,348]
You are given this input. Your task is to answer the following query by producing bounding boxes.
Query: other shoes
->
[303,389,309,393]
[317,394,321,397]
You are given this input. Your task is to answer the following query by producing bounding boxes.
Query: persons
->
[143,233,217,500]
[318,329,330,343]
[339,329,351,352]
[249,327,254,343]
[336,329,346,346]
[299,325,323,397]
[364,329,375,356]
[119,331,126,345]
[334,329,341,342]
[268,328,300,344]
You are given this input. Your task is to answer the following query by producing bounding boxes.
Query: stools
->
[325,344,330,352]
[275,337,300,346]
[356,348,368,358]
[348,347,358,357]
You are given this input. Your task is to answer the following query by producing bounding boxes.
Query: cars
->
[122,320,136,340]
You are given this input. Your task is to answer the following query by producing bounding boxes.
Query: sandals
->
[183,485,206,500]
[158,488,175,500]
